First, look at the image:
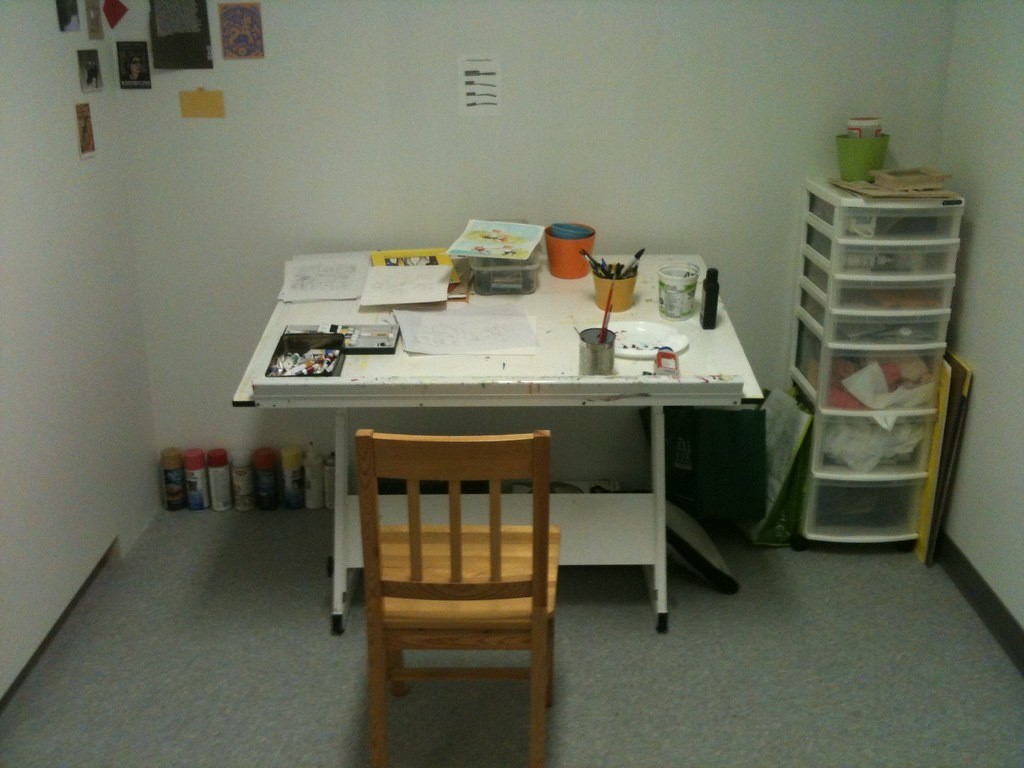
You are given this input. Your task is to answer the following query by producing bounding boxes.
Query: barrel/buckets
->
[545,223,596,279]
[836,134,891,182]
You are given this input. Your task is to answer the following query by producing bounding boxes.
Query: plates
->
[583,321,689,359]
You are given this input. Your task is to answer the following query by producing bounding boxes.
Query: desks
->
[231,250,763,635]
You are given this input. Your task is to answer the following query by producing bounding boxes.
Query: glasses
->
[131,60,142,65]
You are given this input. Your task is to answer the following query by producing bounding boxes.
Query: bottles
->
[322,452,335,509]
[656,347,678,375]
[302,441,323,509]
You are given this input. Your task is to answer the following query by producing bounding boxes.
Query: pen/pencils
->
[596,277,615,344]
[579,245,647,278]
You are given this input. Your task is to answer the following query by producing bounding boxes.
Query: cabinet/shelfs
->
[780,177,964,552]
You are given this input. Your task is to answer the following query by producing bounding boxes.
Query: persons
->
[121,54,149,81]
[79,104,95,154]
[86,61,98,88]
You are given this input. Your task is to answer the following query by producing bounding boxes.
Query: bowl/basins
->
[551,223,593,239]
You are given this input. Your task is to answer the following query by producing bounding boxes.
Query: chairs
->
[354,427,562,768]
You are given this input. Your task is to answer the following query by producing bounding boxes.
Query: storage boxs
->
[467,217,540,295]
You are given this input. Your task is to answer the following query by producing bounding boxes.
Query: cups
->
[579,328,616,374]
[658,263,700,321]
[593,264,639,311]
